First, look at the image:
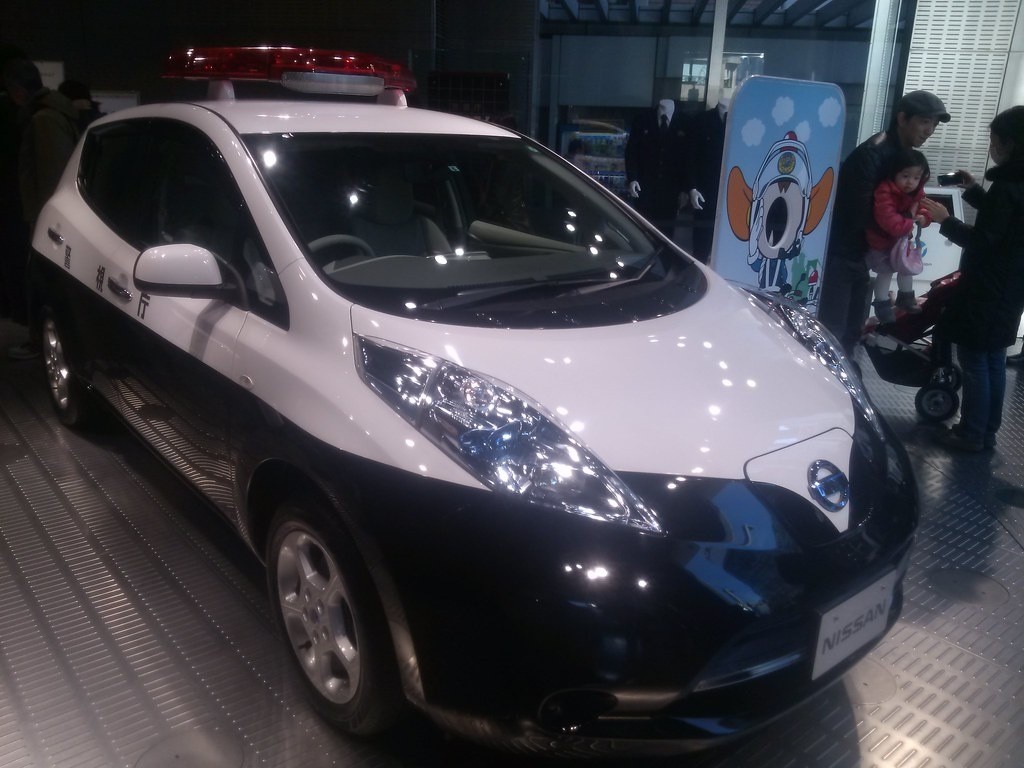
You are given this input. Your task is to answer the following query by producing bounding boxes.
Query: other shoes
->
[8,342,40,360]
[952,424,997,446]
[939,429,984,451]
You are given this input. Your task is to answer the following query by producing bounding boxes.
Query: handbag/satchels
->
[890,220,923,275]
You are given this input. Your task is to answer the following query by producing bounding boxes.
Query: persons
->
[869,150,932,323]
[819,91,951,373]
[624,98,688,239]
[922,105,1024,445]
[686,99,728,264]
[0,41,106,359]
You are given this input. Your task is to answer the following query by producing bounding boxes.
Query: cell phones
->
[938,173,964,187]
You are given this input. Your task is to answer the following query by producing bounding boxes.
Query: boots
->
[895,290,922,314]
[874,298,896,324]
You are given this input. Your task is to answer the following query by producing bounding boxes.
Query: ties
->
[661,115,667,129]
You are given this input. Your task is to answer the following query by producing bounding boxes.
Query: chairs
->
[352,176,454,263]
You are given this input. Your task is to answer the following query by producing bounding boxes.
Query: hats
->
[900,90,951,123]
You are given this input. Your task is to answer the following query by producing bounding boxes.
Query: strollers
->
[858,269,964,421]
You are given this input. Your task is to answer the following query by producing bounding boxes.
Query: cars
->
[22,44,926,763]
